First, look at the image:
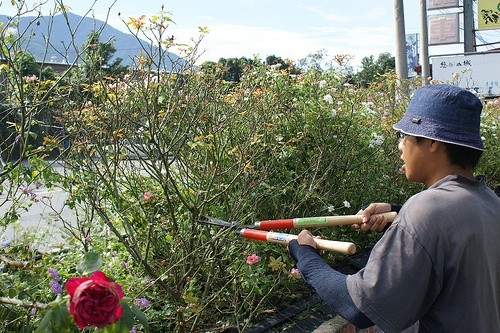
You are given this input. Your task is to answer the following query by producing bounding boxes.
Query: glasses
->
[397,132,409,140]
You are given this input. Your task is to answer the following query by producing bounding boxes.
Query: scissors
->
[197,211,399,256]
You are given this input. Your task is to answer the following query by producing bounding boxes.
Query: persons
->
[286,85,500,333]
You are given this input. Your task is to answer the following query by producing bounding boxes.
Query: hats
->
[393,84,486,152]
